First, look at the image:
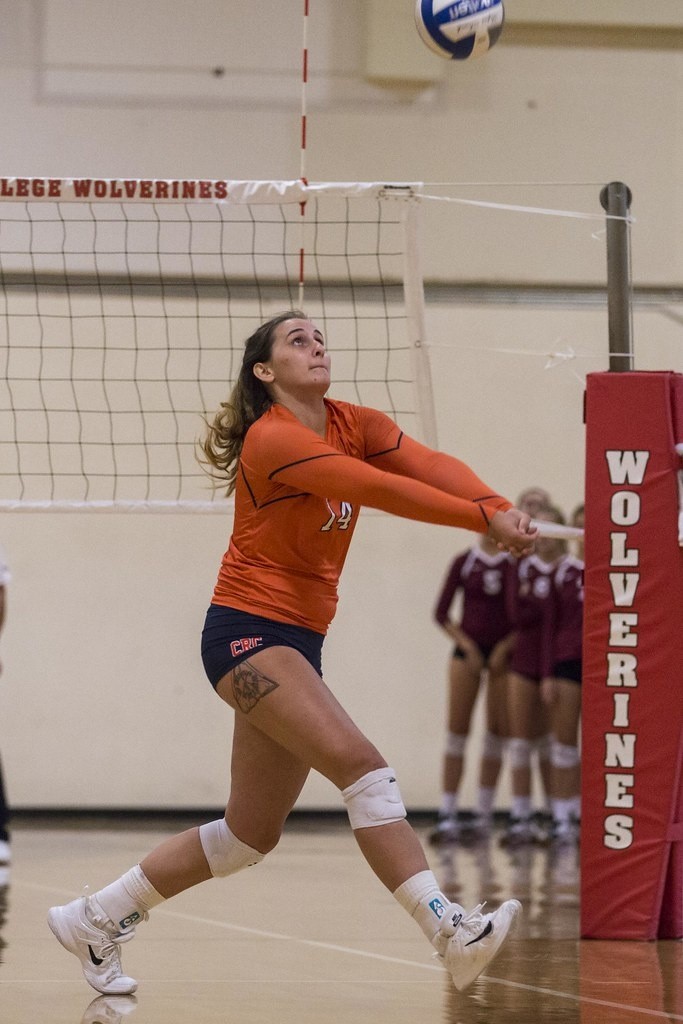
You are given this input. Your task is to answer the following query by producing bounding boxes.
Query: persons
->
[427,488,584,847]
[46,312,538,997]
[0,559,16,864]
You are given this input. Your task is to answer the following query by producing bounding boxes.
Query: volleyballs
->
[414,0,504,60]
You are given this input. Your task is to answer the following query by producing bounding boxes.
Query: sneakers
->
[426,814,583,854]
[429,900,518,993]
[43,898,138,995]
